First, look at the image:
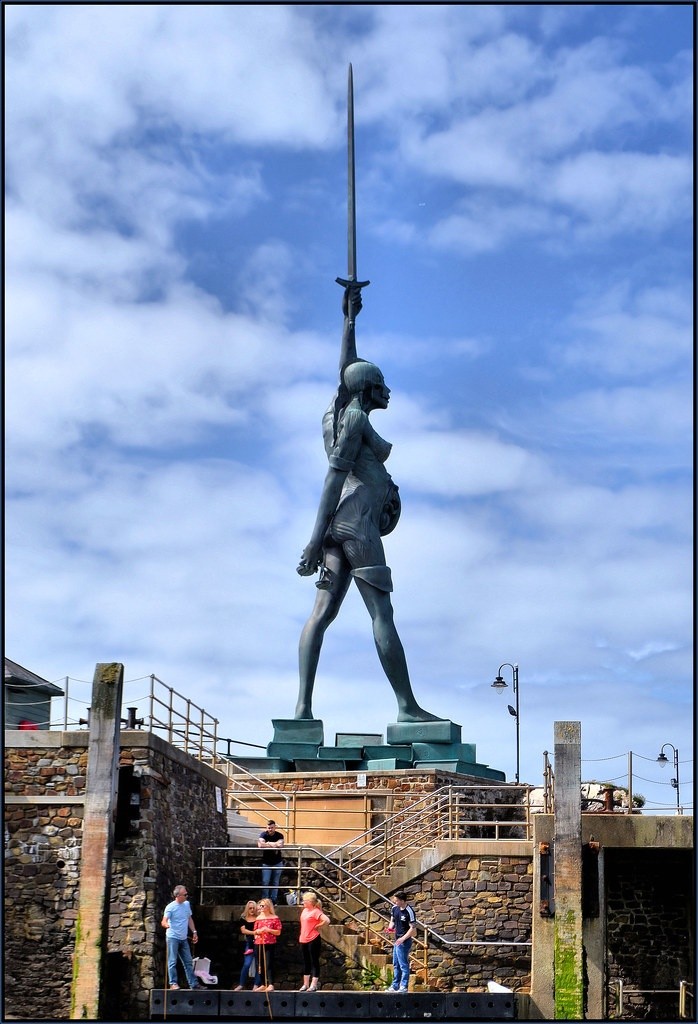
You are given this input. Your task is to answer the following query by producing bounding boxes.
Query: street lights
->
[490,662,520,783]
[657,743,680,814]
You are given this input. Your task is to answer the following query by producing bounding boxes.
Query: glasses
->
[260,905,265,908]
[179,893,188,897]
[250,907,255,909]
[302,900,307,902]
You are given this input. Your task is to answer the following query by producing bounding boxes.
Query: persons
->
[257,820,284,907]
[299,892,330,993]
[161,885,206,988]
[385,890,417,992]
[234,901,262,991]
[253,898,282,991]
[290,361,453,722]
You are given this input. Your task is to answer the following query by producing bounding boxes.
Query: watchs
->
[193,930,197,934]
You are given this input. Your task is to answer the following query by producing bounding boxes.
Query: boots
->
[300,974,310,991]
[306,976,318,992]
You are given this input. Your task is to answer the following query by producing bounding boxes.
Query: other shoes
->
[233,984,245,991]
[385,986,407,992]
[255,985,274,993]
[253,984,258,992]
[191,984,208,990]
[169,983,180,990]
[273,900,277,906]
